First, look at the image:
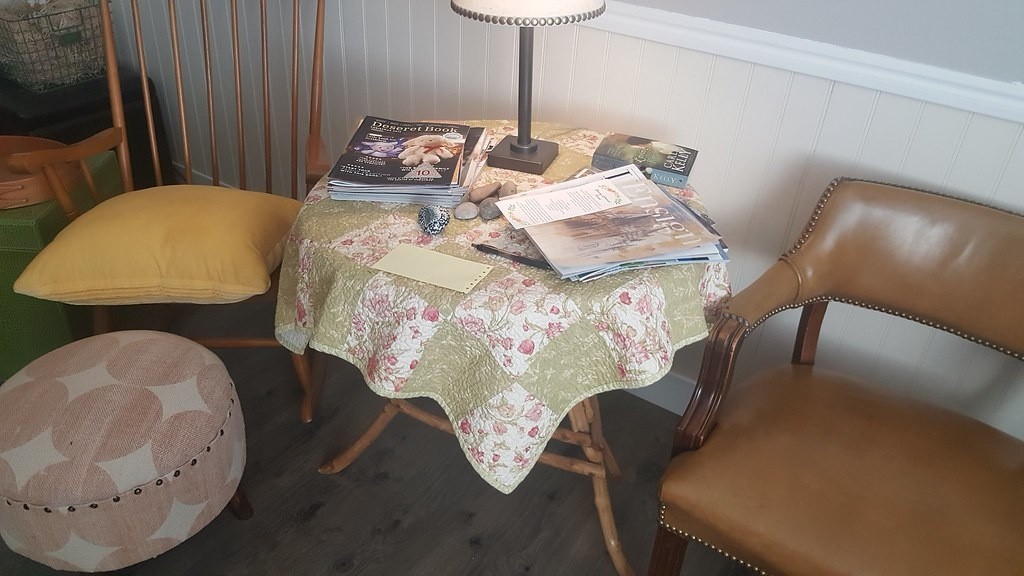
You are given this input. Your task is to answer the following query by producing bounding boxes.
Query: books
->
[326,115,498,213]
[590,131,698,188]
[495,163,730,285]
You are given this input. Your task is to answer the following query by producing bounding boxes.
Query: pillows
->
[12,185,303,307]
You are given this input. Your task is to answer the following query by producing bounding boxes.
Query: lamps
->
[449,0,607,176]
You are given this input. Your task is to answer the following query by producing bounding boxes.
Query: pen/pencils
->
[471,243,553,271]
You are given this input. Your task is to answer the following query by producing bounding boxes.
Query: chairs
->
[5,0,331,424]
[643,174,1023,576]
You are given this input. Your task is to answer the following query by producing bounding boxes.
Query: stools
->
[0,328,247,574]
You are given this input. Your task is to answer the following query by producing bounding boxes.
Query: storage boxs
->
[0,2,114,92]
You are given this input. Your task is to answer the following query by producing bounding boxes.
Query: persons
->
[628,136,685,157]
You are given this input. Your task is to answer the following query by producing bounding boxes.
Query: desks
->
[299,118,727,576]
